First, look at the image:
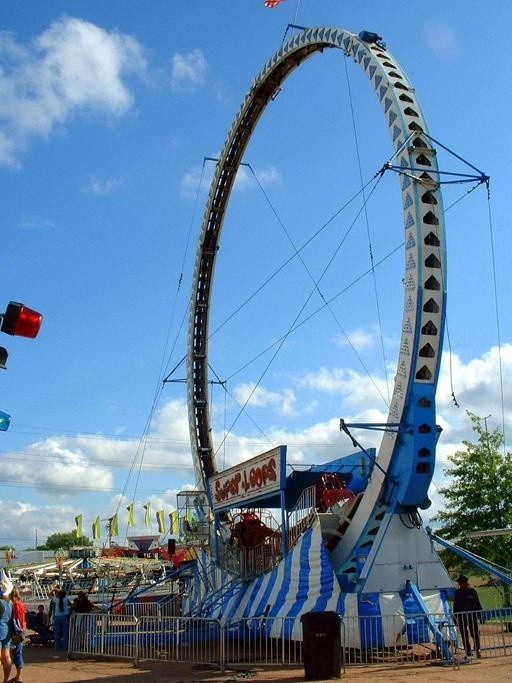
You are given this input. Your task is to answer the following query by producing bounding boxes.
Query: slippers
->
[8,678,23,683]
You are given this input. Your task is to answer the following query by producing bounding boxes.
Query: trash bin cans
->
[300,611,344,680]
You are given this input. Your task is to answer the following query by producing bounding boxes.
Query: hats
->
[455,575,468,582]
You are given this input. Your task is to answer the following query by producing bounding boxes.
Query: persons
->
[1,589,14,683]
[9,585,27,683]
[34,585,96,651]
[452,573,484,660]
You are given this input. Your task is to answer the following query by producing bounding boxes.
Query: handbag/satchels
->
[12,632,25,645]
[9,618,22,635]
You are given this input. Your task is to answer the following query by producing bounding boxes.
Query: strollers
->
[25,609,55,647]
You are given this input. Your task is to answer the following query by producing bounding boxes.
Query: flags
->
[75,499,180,538]
[264,0,282,7]
